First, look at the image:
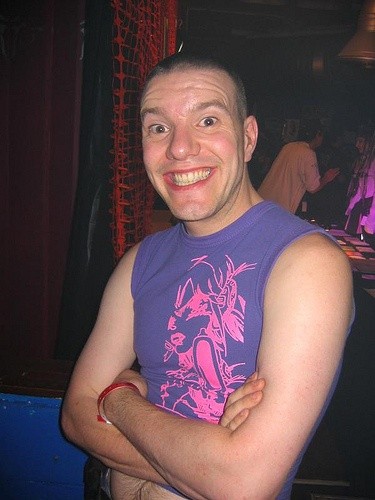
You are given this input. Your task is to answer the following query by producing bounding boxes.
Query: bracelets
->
[96,381,141,425]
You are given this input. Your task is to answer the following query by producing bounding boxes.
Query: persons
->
[254,119,340,215]
[249,115,288,186]
[344,133,375,242]
[57,51,355,499]
